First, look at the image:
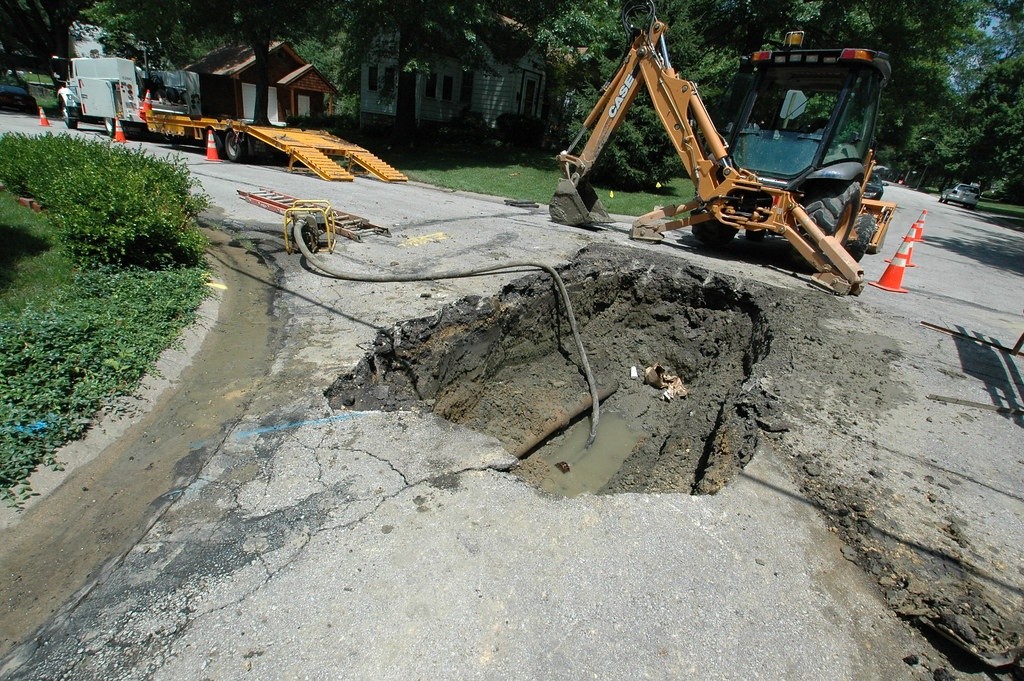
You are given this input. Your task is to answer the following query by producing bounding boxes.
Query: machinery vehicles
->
[548,2,898,295]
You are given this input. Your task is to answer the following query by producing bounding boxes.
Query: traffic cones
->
[204,130,221,163]
[901,209,927,242]
[114,115,127,143]
[139,91,153,112]
[868,236,911,293]
[885,223,917,266]
[38,107,50,127]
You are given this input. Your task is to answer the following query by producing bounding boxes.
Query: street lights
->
[913,137,937,191]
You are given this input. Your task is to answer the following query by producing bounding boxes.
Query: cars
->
[862,172,889,200]
[0,84,37,113]
[938,182,981,211]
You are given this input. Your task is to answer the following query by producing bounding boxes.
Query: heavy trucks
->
[50,22,412,185]
